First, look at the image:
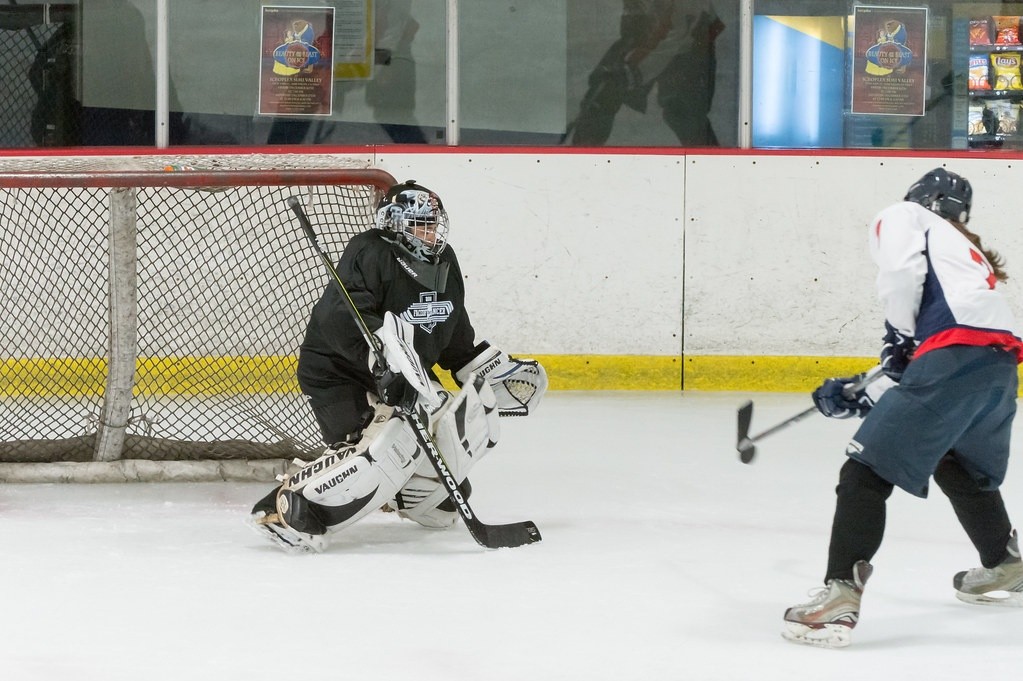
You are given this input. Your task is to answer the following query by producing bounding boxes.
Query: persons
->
[781,165,1023,647]
[29,0,239,144]
[557,0,726,146]
[249,180,547,555]
[266,0,431,144]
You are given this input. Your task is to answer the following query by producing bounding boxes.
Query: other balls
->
[740,446,756,464]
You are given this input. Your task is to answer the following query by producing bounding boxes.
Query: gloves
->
[813,373,874,419]
[878,319,917,381]
[585,62,639,98]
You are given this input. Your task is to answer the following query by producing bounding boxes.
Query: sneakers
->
[248,510,305,554]
[781,559,874,648]
[386,499,453,528]
[953,529,1023,609]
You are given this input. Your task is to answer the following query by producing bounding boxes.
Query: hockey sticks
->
[736,362,883,456]
[288,195,543,550]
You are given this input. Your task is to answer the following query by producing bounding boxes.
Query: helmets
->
[374,180,448,265]
[904,168,972,225]
